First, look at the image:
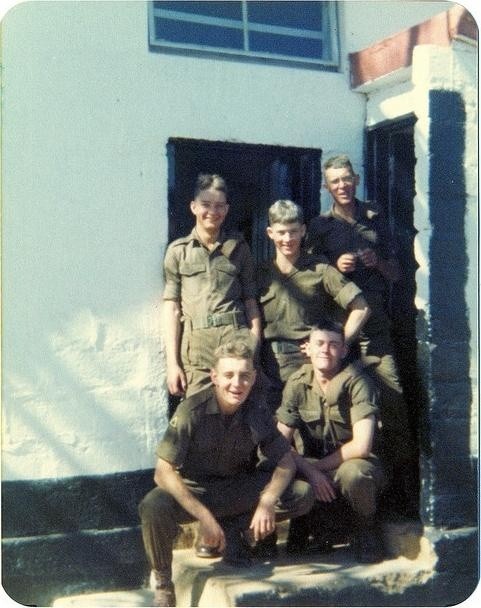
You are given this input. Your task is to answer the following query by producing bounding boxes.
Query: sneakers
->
[152,585,175,607]
[194,522,386,568]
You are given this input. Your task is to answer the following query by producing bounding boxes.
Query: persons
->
[245,198,374,405]
[135,337,299,607]
[159,170,264,562]
[273,319,385,564]
[305,155,419,522]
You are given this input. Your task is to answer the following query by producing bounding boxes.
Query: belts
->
[183,311,245,331]
[272,341,301,354]
